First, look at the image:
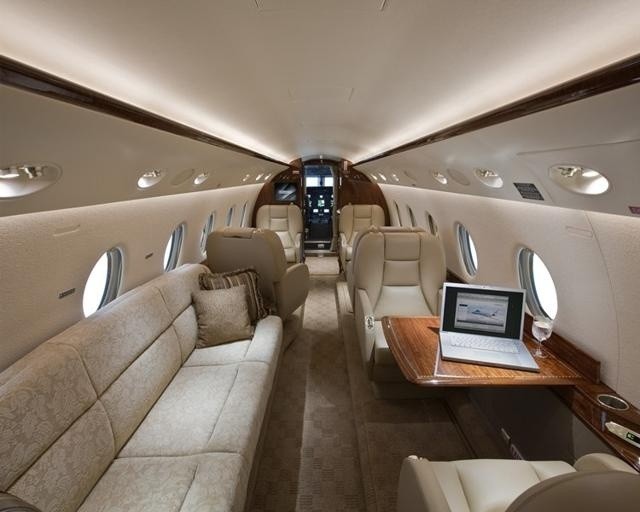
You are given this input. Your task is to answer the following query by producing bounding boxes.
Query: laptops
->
[439,282,540,373]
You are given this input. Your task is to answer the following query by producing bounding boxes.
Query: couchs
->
[0,263,284,512]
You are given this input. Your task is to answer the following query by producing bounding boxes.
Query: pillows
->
[192,284,255,349]
[197,266,272,322]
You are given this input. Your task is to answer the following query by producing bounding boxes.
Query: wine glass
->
[531,316,554,359]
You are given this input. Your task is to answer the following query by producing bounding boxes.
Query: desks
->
[381,316,583,387]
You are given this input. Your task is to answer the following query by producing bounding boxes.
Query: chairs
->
[202,204,310,355]
[338,204,448,401]
[395,453,640,512]
